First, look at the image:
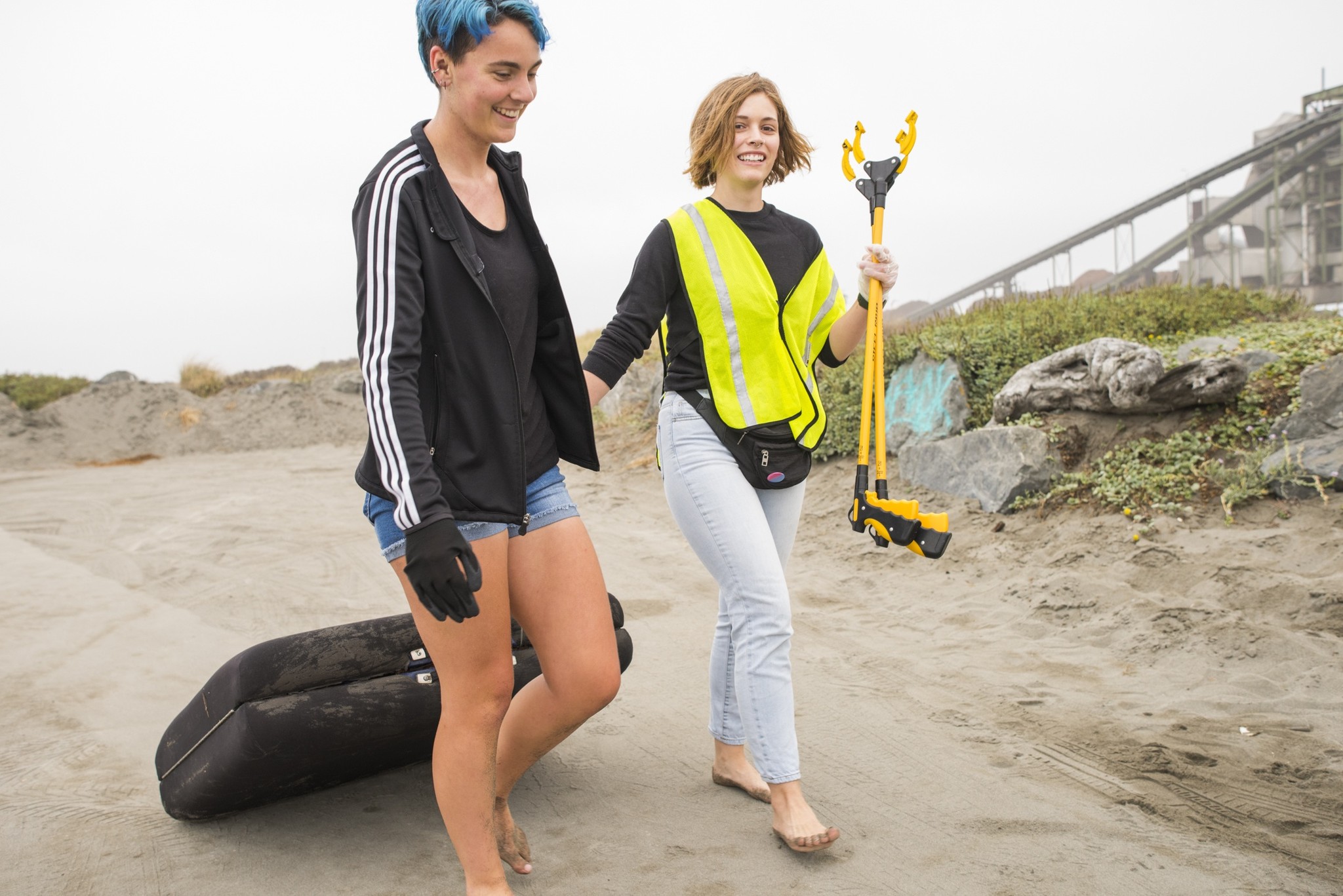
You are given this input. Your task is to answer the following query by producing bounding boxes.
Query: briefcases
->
[149,594,633,825]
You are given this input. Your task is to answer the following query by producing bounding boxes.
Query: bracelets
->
[857,293,887,311]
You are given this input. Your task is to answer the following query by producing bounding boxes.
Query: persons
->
[347,0,619,896]
[585,75,902,861]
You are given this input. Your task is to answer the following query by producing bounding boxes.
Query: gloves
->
[403,519,483,622]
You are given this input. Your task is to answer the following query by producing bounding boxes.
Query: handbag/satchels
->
[695,398,813,490]
